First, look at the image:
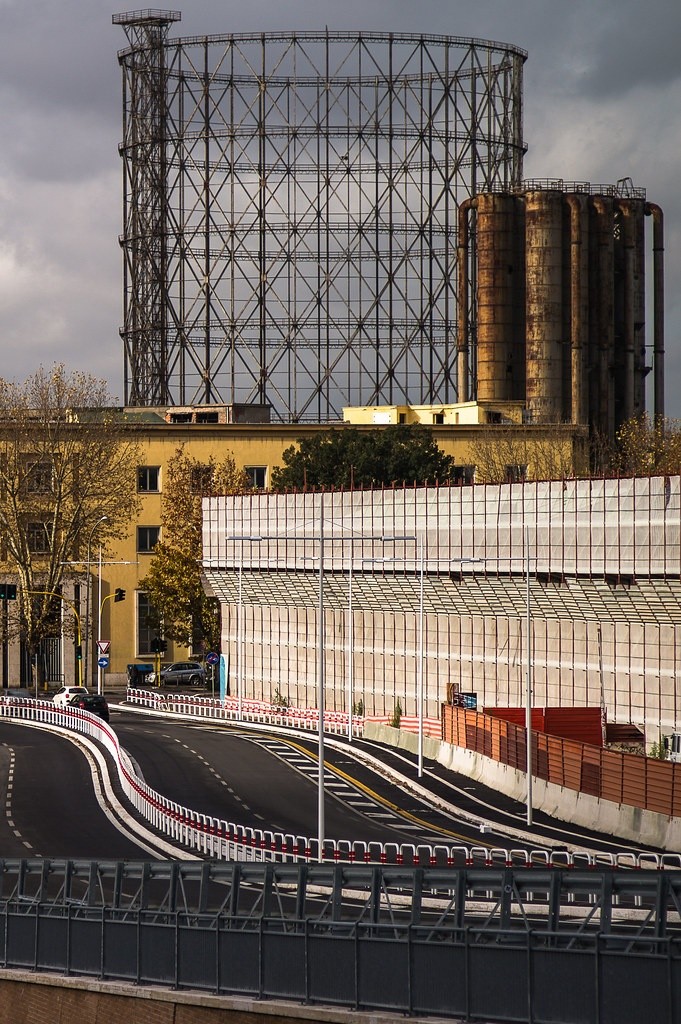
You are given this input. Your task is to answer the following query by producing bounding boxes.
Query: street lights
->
[361,533,480,777]
[461,526,532,826]
[58,544,140,696]
[84,516,107,688]
[225,492,417,865]
[299,540,403,742]
[196,542,285,721]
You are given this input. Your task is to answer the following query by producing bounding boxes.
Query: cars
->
[51,686,89,707]
[145,662,206,687]
[64,694,110,723]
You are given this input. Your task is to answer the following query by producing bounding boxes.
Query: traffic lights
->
[121,590,126,600]
[77,646,82,660]
[0,584,17,600]
[159,639,167,652]
[150,640,156,652]
